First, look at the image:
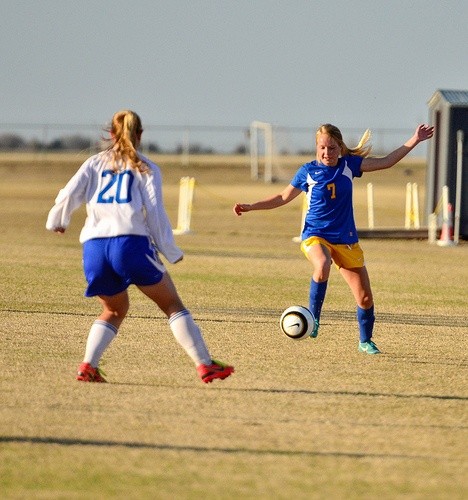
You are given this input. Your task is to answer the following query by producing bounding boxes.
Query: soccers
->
[280,305,316,340]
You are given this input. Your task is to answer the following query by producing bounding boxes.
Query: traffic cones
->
[439,204,454,242]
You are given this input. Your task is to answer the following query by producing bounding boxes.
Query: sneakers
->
[196,359,235,384]
[76,362,107,383]
[309,318,319,338]
[358,340,381,354]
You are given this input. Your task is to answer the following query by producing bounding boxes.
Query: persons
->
[45,111,235,384]
[233,122,435,355]
